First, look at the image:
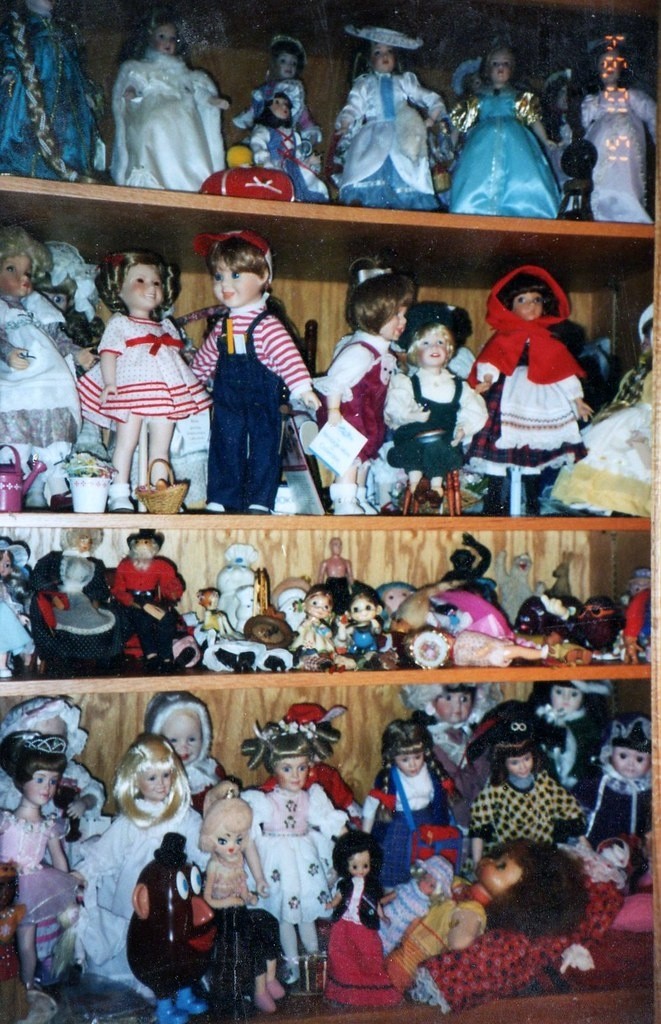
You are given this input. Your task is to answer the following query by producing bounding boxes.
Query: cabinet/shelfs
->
[0,170,653,992]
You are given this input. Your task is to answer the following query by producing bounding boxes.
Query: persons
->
[0,679,652,1024]
[0,1,656,226]
[0,224,654,678]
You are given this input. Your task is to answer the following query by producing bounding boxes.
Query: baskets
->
[134,458,189,514]
